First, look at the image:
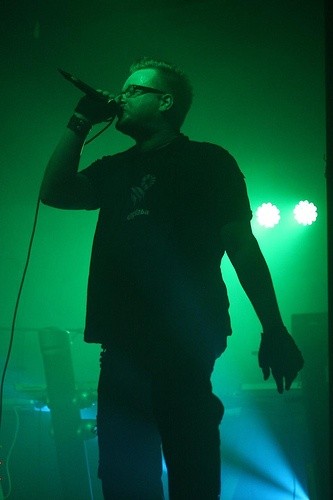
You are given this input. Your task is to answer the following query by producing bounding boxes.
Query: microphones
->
[57,68,123,116]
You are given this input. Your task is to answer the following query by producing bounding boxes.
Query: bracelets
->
[67,114,92,140]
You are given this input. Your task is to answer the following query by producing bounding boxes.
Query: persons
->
[40,59,306,499]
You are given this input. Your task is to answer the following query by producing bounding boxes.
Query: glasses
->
[114,84,164,101]
